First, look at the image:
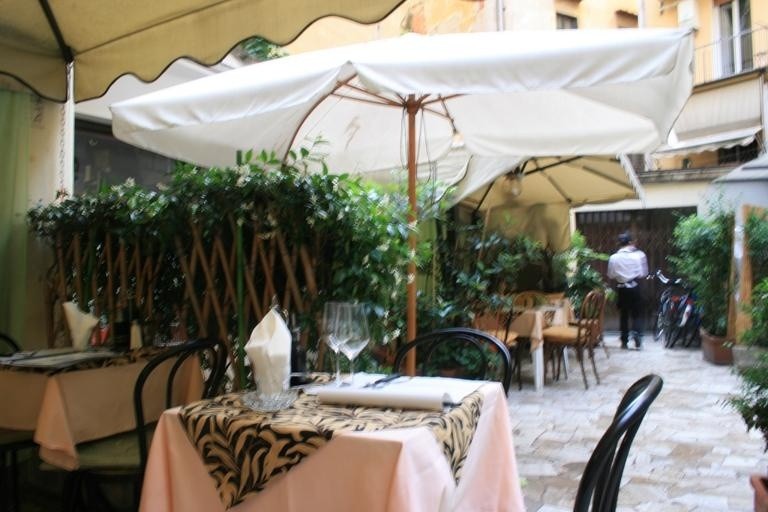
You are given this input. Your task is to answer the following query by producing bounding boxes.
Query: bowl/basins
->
[241,389,298,414]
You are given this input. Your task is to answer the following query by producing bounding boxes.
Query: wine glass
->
[321,301,370,386]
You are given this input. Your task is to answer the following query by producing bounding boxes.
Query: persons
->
[609,224,651,354]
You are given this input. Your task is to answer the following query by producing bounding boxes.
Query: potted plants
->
[664,178,768,512]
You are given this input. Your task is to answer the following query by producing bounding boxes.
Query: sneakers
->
[620,332,641,349]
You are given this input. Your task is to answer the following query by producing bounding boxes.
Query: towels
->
[62,301,99,349]
[243,308,292,394]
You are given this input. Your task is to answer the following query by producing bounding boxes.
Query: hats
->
[618,231,632,245]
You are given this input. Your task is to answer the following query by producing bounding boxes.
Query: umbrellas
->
[470,149,645,274]
[1,0,409,103]
[107,19,699,374]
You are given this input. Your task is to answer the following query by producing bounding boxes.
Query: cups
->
[68,327,88,352]
[249,350,293,391]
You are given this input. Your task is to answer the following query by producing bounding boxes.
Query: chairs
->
[491,287,611,390]
[390,328,512,400]
[61,336,228,512]
[2,334,42,510]
[574,373,664,512]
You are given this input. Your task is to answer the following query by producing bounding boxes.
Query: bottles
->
[112,294,143,349]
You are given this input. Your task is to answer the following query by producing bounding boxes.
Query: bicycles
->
[653,270,705,349]
